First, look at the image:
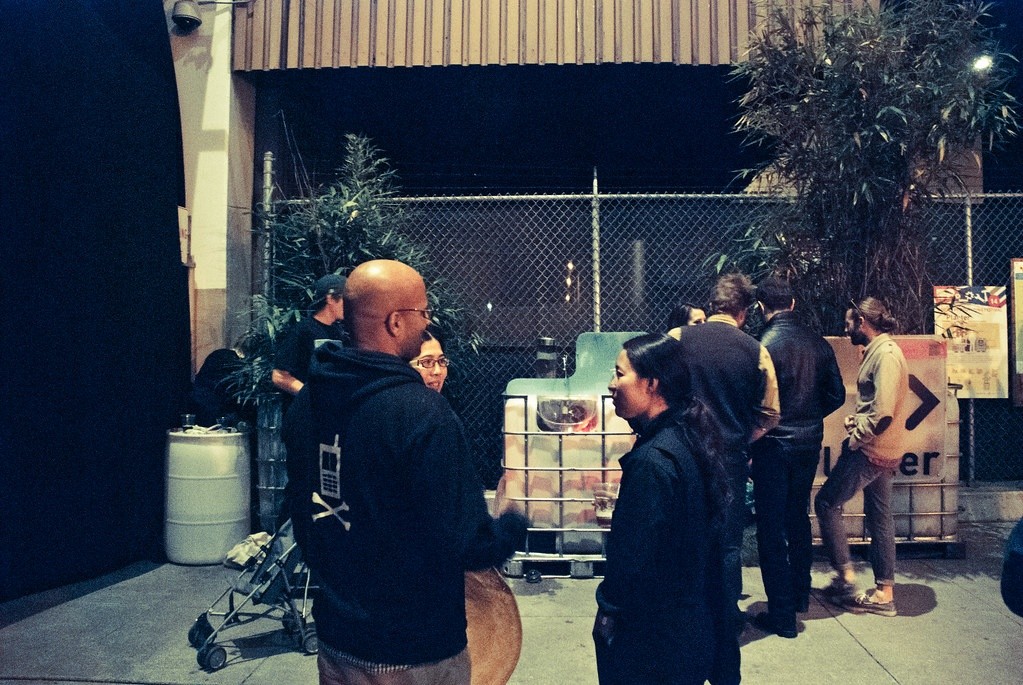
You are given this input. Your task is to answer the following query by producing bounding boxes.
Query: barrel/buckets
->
[165,428,251,565]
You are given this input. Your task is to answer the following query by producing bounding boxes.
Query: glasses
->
[384,303,435,324]
[848,297,866,320]
[410,355,450,368]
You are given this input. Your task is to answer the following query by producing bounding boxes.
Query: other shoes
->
[796,593,810,612]
[755,611,798,639]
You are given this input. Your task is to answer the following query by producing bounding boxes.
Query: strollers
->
[186,515,317,672]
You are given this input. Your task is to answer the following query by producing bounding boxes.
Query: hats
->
[307,274,347,309]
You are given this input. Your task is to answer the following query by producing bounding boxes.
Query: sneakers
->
[824,576,864,599]
[841,594,897,617]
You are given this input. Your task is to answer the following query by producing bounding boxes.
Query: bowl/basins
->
[537,400,597,432]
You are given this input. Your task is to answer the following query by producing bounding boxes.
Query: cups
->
[592,483,619,526]
[217,417,227,429]
[182,415,196,431]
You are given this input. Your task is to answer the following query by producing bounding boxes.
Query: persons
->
[272,275,347,535]
[591,333,732,685]
[407,325,449,392]
[280,260,528,685]
[751,279,846,638]
[814,297,909,616]
[665,273,781,643]
